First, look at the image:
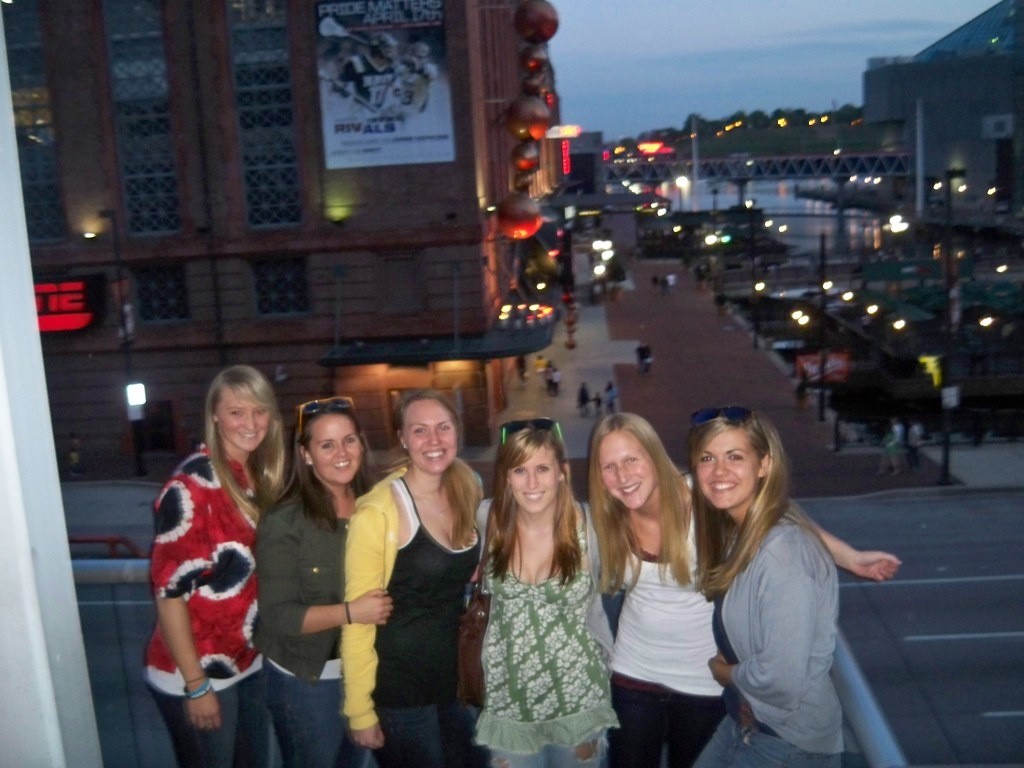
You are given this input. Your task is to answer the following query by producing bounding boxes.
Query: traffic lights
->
[125,380,147,407]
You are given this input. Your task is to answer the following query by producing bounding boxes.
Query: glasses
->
[690,405,753,422]
[500,418,564,444]
[298,396,355,438]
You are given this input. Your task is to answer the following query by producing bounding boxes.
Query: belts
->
[723,688,781,739]
[611,671,672,692]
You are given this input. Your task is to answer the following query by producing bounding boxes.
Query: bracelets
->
[183,674,212,699]
[344,601,352,625]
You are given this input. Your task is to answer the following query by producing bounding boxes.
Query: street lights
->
[95,206,148,477]
[939,165,969,486]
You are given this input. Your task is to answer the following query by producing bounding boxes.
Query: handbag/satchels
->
[457,593,490,707]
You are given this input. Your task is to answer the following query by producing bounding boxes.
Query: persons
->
[588,412,902,768]
[339,389,485,768]
[146,364,288,767]
[253,395,393,768]
[469,418,623,768]
[687,404,845,768]
[532,340,653,418]
[564,304,577,349]
[651,270,677,296]
